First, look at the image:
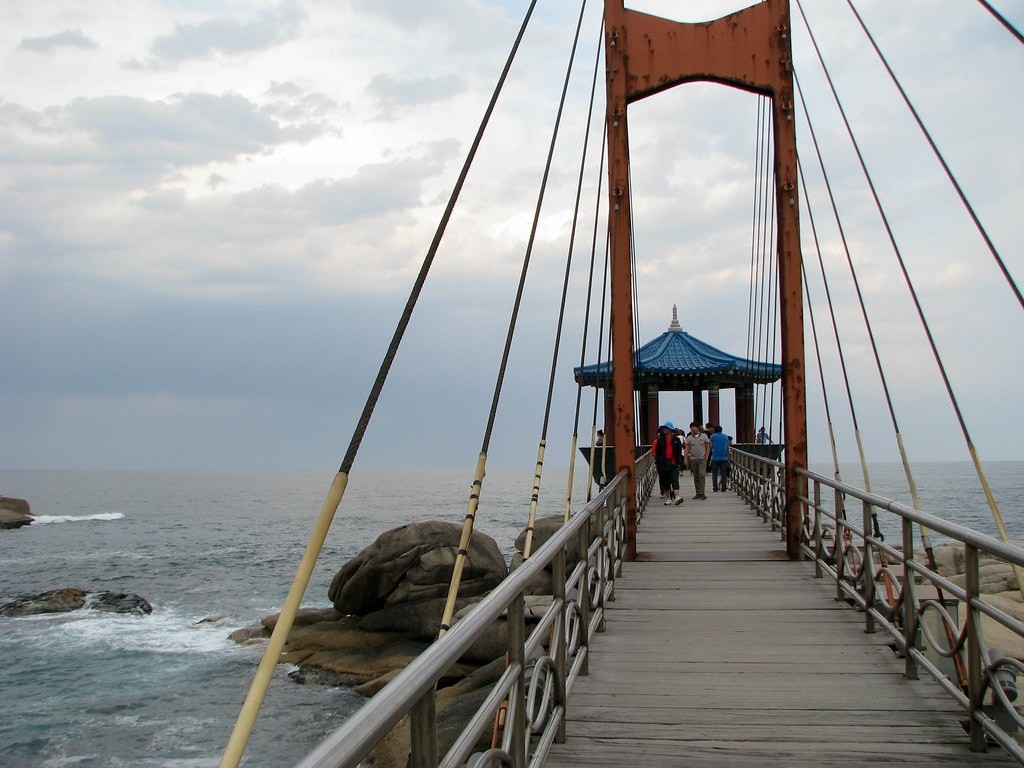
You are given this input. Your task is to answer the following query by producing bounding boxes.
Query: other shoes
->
[712,487,717,492]
[660,492,666,498]
[693,494,707,500]
[721,488,726,492]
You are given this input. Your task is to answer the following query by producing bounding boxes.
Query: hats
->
[660,422,674,431]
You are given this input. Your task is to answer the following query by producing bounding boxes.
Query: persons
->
[596,430,604,446]
[757,427,774,444]
[675,428,686,476]
[704,423,733,492]
[685,422,711,500]
[651,421,683,505]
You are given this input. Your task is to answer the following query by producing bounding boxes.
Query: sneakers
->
[664,498,672,505]
[674,497,683,505]
